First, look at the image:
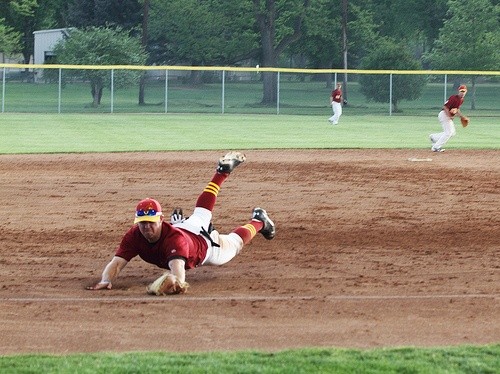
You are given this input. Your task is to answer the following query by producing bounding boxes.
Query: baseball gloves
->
[461,116,470,127]
[343,100,348,104]
[145,273,189,296]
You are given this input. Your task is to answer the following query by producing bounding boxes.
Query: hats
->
[337,82,341,86]
[458,85,467,92]
[134,197,162,224]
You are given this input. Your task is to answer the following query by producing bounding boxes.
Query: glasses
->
[136,210,158,217]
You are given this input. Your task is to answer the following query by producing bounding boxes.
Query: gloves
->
[200,222,220,248]
[170,208,189,225]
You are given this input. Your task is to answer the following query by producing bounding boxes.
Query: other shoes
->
[333,122,338,125]
[328,119,333,124]
[430,134,435,143]
[431,147,445,152]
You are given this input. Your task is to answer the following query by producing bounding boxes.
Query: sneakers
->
[252,208,275,240]
[216,151,246,174]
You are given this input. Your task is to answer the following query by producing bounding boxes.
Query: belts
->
[442,109,445,110]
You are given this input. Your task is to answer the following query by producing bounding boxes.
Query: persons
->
[88,152,276,295]
[429,85,469,152]
[328,82,347,124]
